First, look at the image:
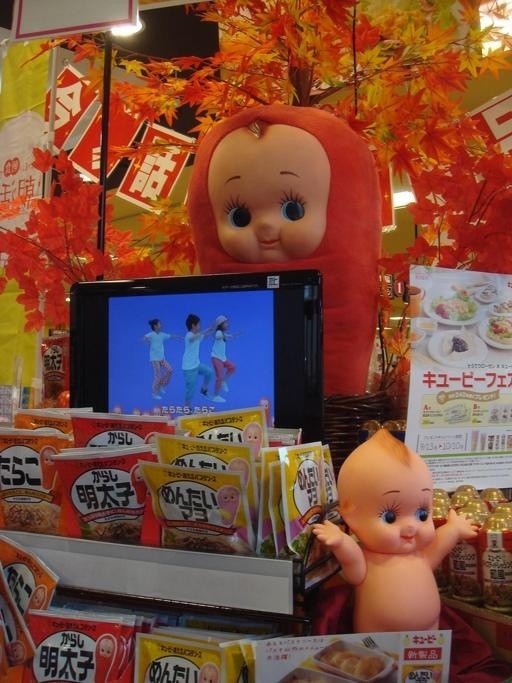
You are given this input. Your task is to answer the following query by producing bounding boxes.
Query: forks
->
[362,636,388,655]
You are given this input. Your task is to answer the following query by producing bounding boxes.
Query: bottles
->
[429,485,512,611]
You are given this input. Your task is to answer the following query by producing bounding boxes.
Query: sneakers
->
[199,387,212,401]
[152,392,164,400]
[159,384,167,394]
[212,395,228,403]
[222,382,229,393]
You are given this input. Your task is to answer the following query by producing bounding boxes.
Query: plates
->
[408,276,511,368]
[313,639,397,682]
[276,666,357,683]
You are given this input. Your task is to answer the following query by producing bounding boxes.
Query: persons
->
[180,313,216,412]
[186,104,383,395]
[209,313,245,404]
[140,318,185,401]
[312,425,478,632]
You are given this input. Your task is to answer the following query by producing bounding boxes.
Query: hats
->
[213,314,228,327]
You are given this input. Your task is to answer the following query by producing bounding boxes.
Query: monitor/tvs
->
[69,269,323,444]
